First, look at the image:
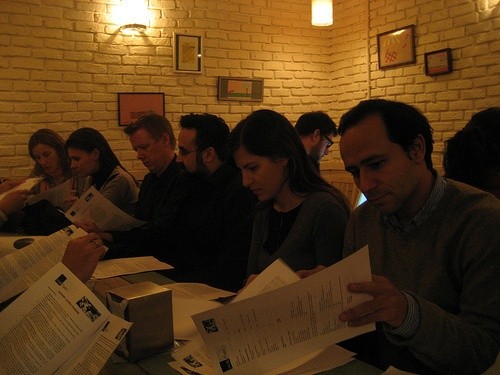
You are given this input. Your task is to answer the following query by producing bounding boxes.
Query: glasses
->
[179,146,197,156]
[309,128,334,149]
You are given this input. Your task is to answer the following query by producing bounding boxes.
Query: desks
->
[80,272,383,375]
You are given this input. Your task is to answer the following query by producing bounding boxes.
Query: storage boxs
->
[105,281,174,363]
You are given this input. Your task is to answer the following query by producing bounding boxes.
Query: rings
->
[92,239,97,248]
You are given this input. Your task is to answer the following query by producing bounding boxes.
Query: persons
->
[0,109,354,299]
[297,98,500,375]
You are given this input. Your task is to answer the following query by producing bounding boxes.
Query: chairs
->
[325,174,359,205]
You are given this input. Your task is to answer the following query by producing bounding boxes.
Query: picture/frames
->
[217,76,265,103]
[117,92,166,127]
[377,23,417,70]
[172,32,203,74]
[424,48,453,76]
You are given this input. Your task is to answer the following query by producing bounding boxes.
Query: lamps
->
[116,9,150,34]
[311,0,334,26]
